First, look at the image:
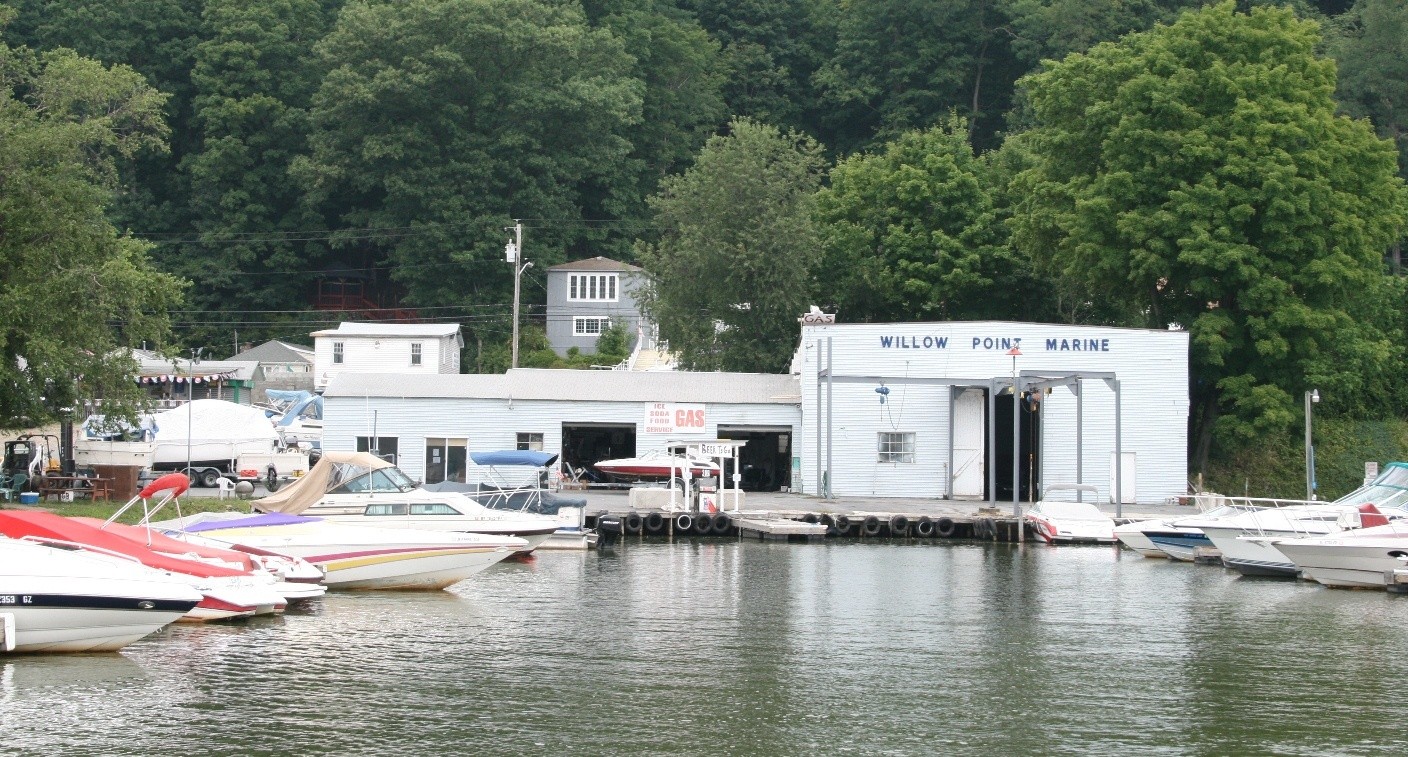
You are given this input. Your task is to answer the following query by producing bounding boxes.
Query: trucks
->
[74,415,321,487]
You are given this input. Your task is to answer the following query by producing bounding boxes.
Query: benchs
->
[40,486,115,492]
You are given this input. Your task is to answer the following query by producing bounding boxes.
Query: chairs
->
[0,473,27,503]
[216,478,236,501]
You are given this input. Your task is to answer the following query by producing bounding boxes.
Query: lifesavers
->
[801,513,818,523]
[694,514,712,534]
[836,516,851,535]
[819,515,835,534]
[712,514,731,533]
[935,516,956,538]
[625,514,643,533]
[892,515,909,536]
[863,514,881,536]
[675,513,693,532]
[645,512,664,533]
[914,515,935,538]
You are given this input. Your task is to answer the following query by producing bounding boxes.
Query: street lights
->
[512,262,534,368]
[1304,389,1320,506]
[1004,343,1024,517]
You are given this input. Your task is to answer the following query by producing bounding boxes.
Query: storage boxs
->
[20,492,39,503]
[563,478,588,489]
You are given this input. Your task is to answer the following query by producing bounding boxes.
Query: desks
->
[43,477,109,504]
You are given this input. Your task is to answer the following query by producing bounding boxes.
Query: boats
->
[0,395,598,655]
[1025,462,1408,593]
[593,443,718,475]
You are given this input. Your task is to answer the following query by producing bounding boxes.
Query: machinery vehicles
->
[1,434,59,494]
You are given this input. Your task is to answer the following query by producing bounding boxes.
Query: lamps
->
[507,394,514,409]
